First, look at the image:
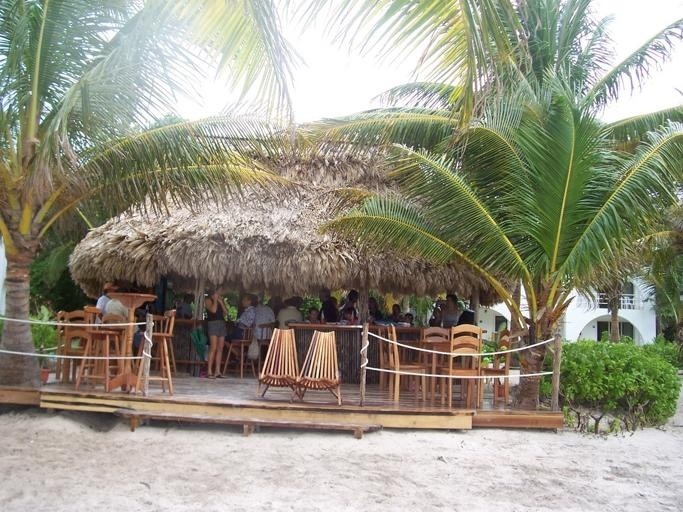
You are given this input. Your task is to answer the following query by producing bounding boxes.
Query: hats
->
[104,282,120,290]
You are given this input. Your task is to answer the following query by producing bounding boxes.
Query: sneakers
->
[231,355,241,364]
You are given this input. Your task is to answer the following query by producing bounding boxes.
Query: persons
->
[228,282,463,342]
[204,281,229,378]
[94,283,196,350]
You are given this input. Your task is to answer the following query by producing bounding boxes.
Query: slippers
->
[208,373,226,379]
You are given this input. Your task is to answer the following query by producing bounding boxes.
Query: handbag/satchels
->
[247,340,258,360]
